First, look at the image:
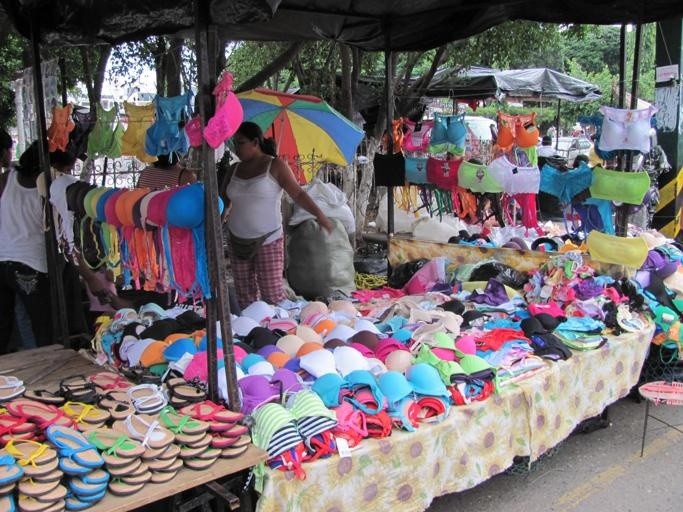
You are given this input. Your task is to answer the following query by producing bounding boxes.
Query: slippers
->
[0,364,251,512]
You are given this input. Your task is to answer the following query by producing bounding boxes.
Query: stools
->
[636,380,682,455]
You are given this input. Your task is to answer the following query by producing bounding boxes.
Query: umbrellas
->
[395,66,603,102]
[218,84,366,186]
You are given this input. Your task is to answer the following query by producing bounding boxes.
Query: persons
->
[98,287,134,312]
[0,139,111,348]
[132,151,199,194]
[217,122,333,313]
